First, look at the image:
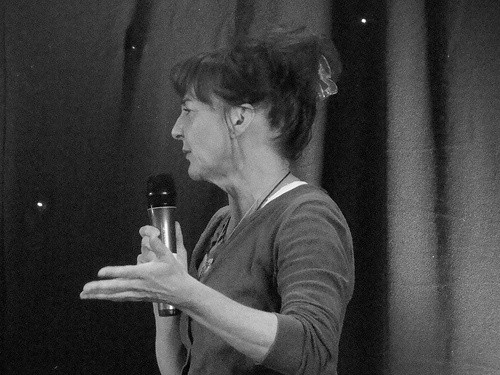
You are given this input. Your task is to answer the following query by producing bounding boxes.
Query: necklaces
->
[223,170,295,240]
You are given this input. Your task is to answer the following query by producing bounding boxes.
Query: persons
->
[79,28,356,375]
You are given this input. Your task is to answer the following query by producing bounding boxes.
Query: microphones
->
[146,173,181,317]
